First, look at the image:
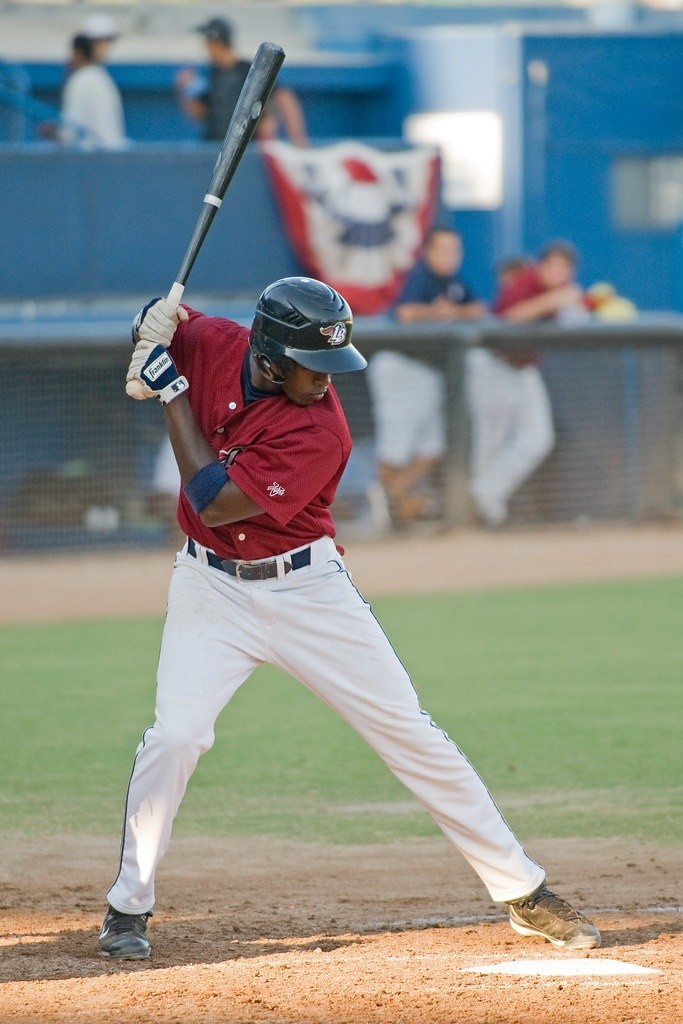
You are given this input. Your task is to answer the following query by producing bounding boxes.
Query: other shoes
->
[375,455,440,538]
[462,477,511,526]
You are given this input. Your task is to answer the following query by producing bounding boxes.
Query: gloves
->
[126,339,189,407]
[132,295,189,349]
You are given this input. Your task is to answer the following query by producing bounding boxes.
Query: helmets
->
[249,277,368,383]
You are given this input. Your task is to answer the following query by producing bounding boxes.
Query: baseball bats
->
[124,41,287,401]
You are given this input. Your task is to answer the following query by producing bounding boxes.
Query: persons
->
[364,226,601,539]
[40,19,128,151]
[175,18,310,147]
[95,278,602,959]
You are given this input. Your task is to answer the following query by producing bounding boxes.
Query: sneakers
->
[508,883,601,950]
[97,903,154,962]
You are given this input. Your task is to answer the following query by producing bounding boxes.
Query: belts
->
[188,537,311,585]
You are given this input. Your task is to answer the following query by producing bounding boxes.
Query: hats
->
[81,16,121,42]
[194,18,233,47]
